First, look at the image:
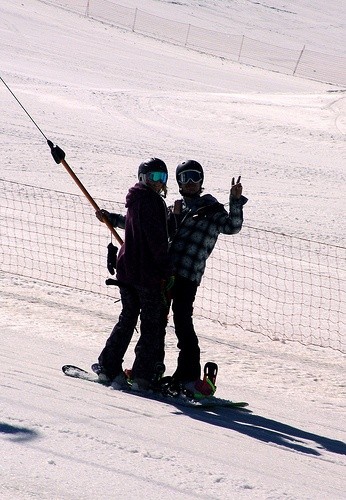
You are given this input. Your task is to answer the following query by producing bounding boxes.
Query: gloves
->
[165,274,175,293]
[107,243,118,275]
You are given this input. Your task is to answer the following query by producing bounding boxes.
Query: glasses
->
[177,170,202,185]
[145,172,167,183]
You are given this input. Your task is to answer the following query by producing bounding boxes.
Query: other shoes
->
[99,360,127,383]
[130,372,158,389]
[166,383,201,397]
[125,369,133,378]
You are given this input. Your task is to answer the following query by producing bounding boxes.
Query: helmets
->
[176,160,204,185]
[138,157,168,184]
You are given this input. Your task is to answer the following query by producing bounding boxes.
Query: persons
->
[98,157,176,391]
[96,160,249,387]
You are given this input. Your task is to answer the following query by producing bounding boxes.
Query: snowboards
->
[60,360,250,410]
